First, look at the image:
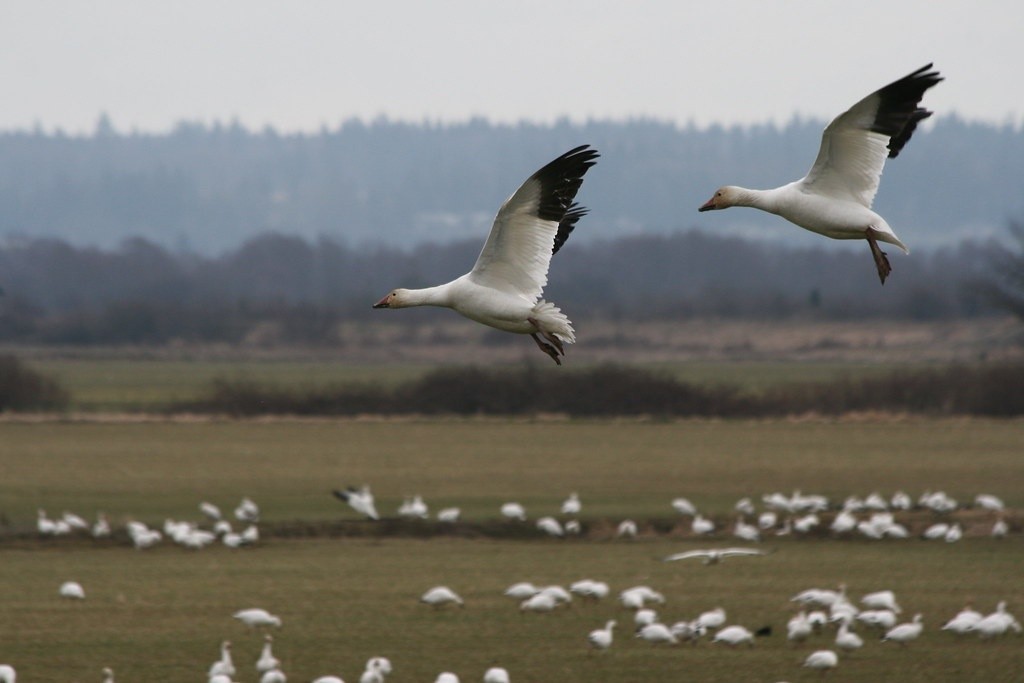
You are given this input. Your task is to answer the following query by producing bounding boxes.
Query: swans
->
[698,61,945,285]
[0,468,1024,683]
[372,144,602,365]
